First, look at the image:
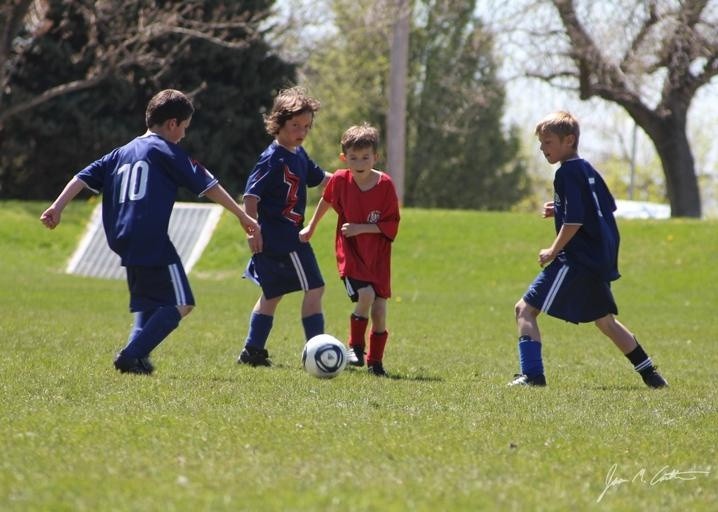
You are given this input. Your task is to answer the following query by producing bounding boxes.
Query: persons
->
[38,88,260,375]
[236,87,334,368]
[505,111,670,389]
[299,123,401,379]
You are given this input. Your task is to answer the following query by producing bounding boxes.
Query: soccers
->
[301,334,347,380]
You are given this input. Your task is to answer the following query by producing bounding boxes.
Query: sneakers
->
[368,361,384,375]
[237,345,271,366]
[348,345,366,366]
[508,372,547,389]
[646,371,667,388]
[113,349,153,374]
